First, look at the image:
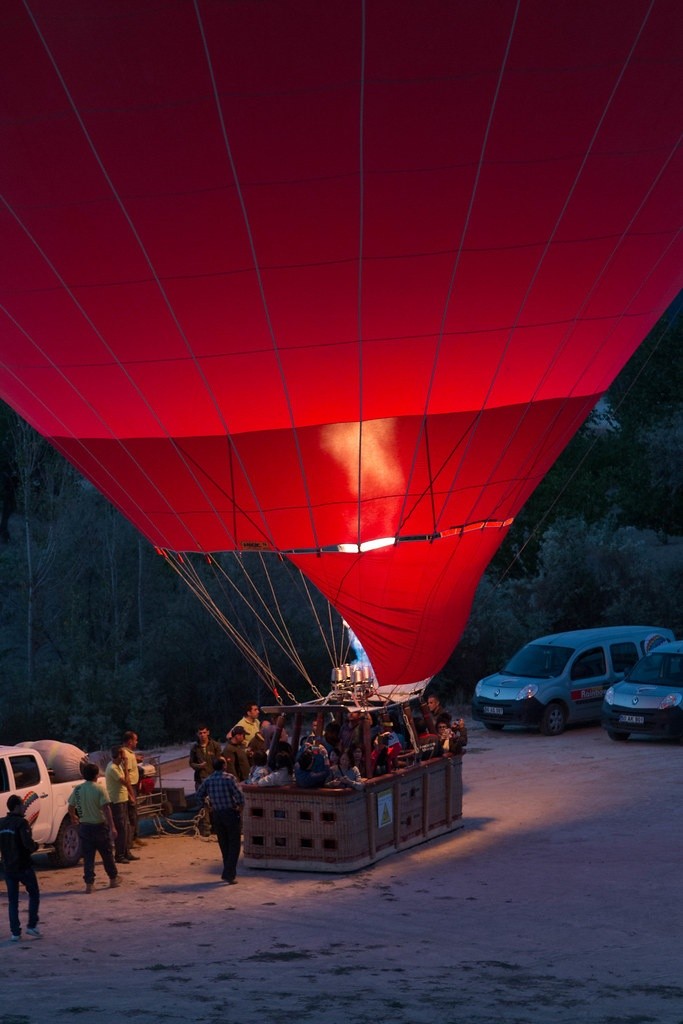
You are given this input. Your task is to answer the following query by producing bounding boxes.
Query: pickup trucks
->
[0,744,108,867]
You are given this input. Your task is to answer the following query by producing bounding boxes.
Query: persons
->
[68,763,123,894]
[248,694,467,845]
[223,726,250,781]
[195,755,244,884]
[227,705,260,747]
[0,794,44,941]
[121,732,148,850]
[105,747,140,864]
[247,720,272,765]
[190,724,223,837]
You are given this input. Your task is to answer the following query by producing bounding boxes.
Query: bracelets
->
[351,781,355,786]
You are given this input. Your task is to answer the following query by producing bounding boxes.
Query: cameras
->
[455,719,460,725]
[308,746,319,750]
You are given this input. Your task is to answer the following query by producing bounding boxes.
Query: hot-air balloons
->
[0,1,683,878]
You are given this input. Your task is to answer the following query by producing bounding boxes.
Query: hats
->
[232,726,250,737]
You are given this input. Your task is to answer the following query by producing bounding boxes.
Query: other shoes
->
[110,876,123,887]
[120,858,130,864]
[228,879,237,884]
[12,933,22,941]
[129,854,140,860]
[26,929,45,938]
[136,838,147,845]
[86,883,96,893]
[133,842,142,849]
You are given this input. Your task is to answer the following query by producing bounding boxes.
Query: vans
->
[604,640,683,743]
[472,622,676,736]
